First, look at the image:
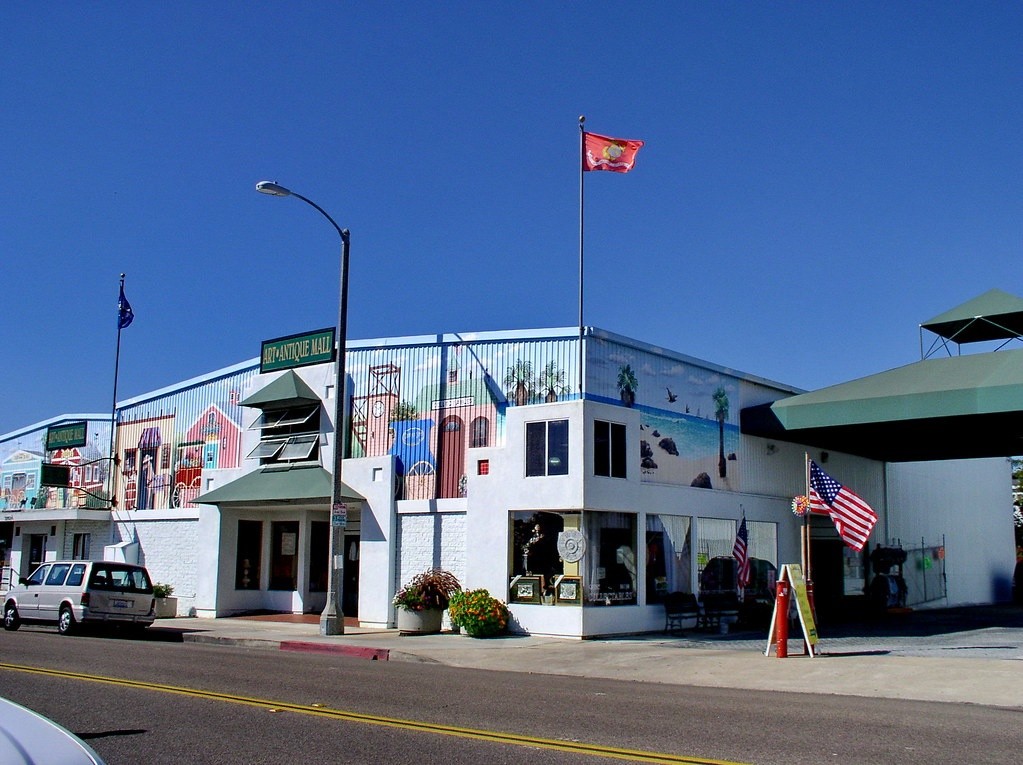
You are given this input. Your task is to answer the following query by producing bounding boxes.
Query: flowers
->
[449,587,508,637]
[392,568,462,606]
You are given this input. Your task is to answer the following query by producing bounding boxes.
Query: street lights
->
[255,179,352,636]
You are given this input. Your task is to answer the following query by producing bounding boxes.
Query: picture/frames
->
[555,575,583,606]
[509,575,540,604]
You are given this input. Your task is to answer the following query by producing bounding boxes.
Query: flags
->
[732,516,751,603]
[582,129,644,174]
[809,459,880,551]
[117,283,136,331]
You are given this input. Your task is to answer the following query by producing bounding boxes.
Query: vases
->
[395,603,443,636]
[460,625,472,635]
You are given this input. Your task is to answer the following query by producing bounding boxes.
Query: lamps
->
[255,181,291,196]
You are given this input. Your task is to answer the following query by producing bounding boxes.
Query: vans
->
[1,560,156,636]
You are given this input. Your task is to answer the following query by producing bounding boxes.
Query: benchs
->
[701,589,748,631]
[663,589,712,637]
